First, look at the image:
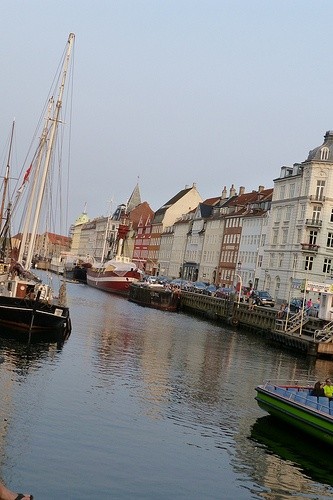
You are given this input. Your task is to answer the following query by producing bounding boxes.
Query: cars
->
[252,290,276,308]
[146,276,249,302]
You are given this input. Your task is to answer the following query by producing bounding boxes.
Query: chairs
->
[266,385,333,415]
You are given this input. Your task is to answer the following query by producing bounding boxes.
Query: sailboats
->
[1,31,75,334]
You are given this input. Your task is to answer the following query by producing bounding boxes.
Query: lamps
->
[238,260,241,267]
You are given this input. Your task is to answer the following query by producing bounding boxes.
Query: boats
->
[254,379,333,443]
[31,249,143,296]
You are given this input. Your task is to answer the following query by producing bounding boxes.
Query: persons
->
[307,297,320,308]
[321,378,333,398]
